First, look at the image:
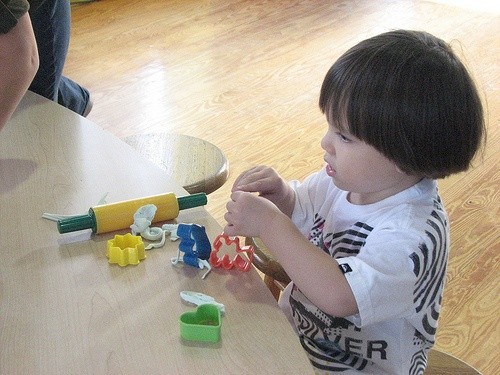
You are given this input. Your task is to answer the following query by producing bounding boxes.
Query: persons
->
[224,29,488,375]
[0,0,93,132]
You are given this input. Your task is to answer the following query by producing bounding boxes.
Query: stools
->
[120,131,229,196]
[245,234,291,303]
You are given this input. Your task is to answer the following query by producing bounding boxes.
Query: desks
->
[1,90,317,372]
